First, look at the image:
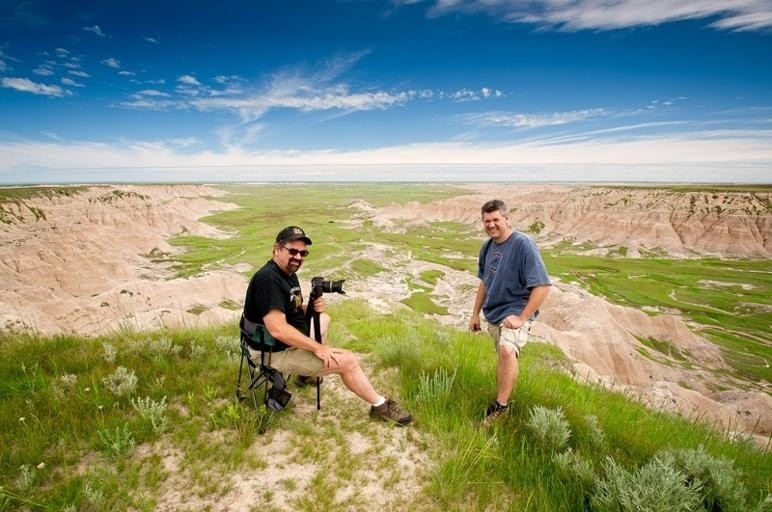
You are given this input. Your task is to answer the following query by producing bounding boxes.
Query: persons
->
[468,199,552,428]
[243,226,413,427]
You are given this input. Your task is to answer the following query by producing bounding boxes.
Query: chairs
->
[236,312,321,435]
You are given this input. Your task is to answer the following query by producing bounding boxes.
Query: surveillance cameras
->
[310,275,347,297]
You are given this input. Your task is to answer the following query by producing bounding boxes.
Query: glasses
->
[282,246,309,257]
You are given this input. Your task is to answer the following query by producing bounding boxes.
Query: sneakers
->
[482,403,509,429]
[294,375,324,386]
[369,397,413,428]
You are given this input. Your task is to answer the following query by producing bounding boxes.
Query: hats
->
[277,225,312,245]
